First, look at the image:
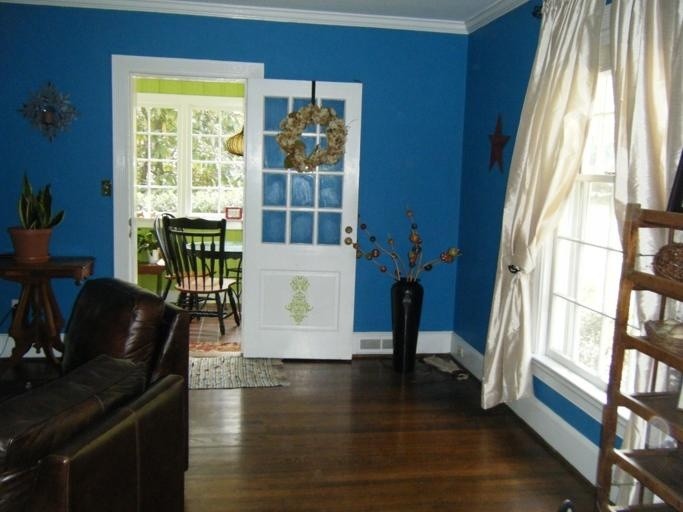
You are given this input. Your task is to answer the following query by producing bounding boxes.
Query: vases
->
[390,278,425,373]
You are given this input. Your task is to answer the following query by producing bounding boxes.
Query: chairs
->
[155,213,244,335]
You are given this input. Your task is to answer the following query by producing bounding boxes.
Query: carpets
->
[188,356,294,390]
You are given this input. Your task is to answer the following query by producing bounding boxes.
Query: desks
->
[138,262,166,296]
[0,255,96,369]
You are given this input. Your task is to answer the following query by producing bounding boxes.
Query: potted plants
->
[8,170,64,265]
[138,228,163,264]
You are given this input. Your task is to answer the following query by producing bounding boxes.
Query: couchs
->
[0,353,189,512]
[56,279,187,381]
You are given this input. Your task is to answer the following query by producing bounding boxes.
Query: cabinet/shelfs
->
[593,202,683,512]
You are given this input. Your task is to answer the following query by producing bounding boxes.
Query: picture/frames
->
[226,207,243,219]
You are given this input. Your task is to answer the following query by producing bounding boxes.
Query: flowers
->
[276,103,348,173]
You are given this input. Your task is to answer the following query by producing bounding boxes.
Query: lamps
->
[226,125,245,156]
